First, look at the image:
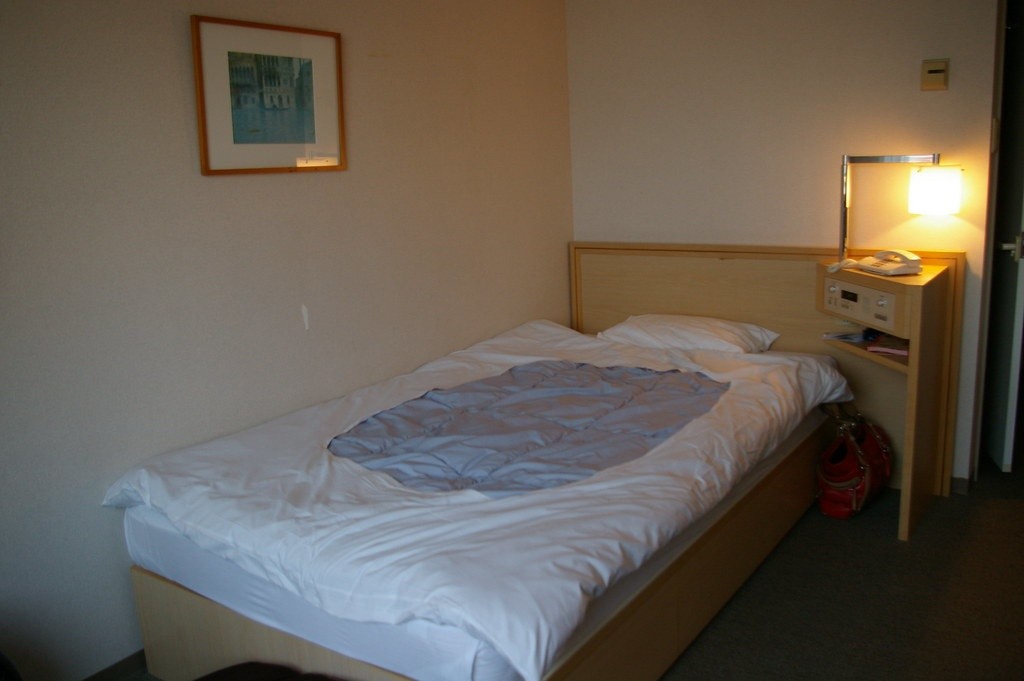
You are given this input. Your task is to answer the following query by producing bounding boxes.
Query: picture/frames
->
[191,15,348,176]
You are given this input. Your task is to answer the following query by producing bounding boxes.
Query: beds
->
[102,240,967,681]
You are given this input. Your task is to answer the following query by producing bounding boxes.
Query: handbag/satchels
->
[815,400,895,520]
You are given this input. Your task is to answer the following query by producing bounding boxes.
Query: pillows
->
[596,313,781,354]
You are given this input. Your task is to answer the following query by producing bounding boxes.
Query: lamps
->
[838,153,965,266]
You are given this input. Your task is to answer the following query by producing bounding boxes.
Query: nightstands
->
[816,263,950,542]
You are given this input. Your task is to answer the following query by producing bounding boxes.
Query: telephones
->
[827,247,924,277]
